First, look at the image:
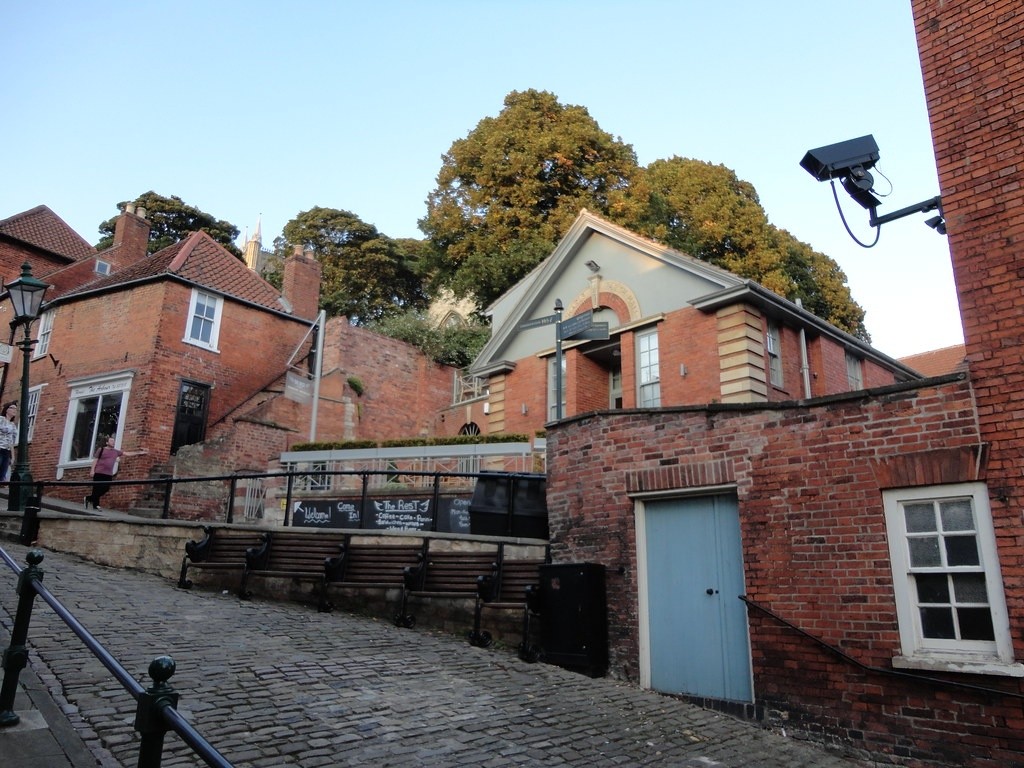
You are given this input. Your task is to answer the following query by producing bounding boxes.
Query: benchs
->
[178,526,552,662]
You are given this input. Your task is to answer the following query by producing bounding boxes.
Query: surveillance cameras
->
[798,134,879,182]
[925,216,942,229]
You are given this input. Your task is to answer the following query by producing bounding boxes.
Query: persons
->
[84,437,148,513]
[0,402,18,487]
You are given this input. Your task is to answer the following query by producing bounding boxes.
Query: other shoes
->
[84,497,88,508]
[94,505,102,512]
